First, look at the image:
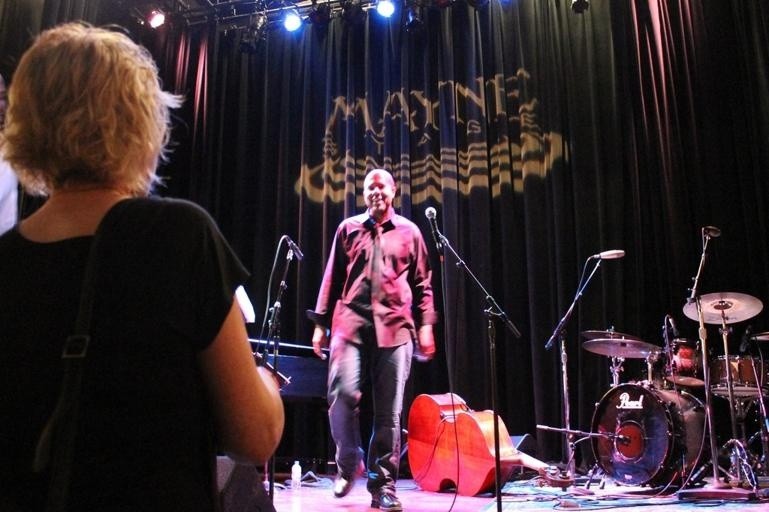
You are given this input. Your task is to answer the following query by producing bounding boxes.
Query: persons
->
[310,167,439,512]
[0,22,288,510]
[0,60,19,235]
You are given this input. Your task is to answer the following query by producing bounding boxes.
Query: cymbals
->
[583,338,663,356]
[685,293,761,324]
[581,328,635,338]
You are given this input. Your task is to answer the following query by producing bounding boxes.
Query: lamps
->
[148,10,165,28]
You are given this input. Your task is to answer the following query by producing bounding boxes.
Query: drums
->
[714,350,761,401]
[592,386,710,489]
[666,338,705,384]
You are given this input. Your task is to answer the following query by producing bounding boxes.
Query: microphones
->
[424,206,445,262]
[701,226,722,237]
[593,250,624,260]
[739,323,756,353]
[284,237,307,264]
[665,316,681,337]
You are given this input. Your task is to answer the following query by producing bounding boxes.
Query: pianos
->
[248,331,330,398]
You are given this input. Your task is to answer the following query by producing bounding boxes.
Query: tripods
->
[673,299,769,491]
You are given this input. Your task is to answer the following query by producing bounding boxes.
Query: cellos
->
[407,393,574,498]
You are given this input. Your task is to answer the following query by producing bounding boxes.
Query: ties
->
[371,227,384,299]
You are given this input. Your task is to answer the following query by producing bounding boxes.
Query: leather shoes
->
[371,492,402,512]
[333,474,354,498]
